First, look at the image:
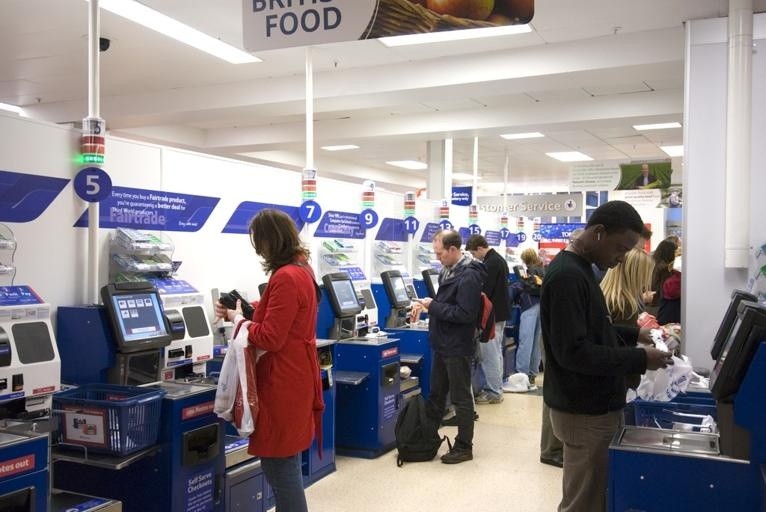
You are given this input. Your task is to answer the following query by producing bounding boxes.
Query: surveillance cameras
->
[99,39,111,52]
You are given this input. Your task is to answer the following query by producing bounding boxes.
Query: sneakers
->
[440,415,457,426]
[541,450,563,467]
[440,447,472,464]
[475,391,503,404]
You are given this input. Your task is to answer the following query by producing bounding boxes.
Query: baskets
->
[51,384,164,457]
[632,391,718,428]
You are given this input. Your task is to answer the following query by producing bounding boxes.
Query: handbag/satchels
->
[478,291,494,343]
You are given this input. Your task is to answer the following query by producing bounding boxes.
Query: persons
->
[540,228,587,468]
[464,232,512,405]
[411,228,486,465]
[631,164,658,189]
[520,247,545,279]
[591,225,680,394]
[539,200,674,512]
[506,278,541,394]
[211,205,329,512]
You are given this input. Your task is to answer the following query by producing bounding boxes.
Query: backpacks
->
[394,396,440,461]
[511,274,542,301]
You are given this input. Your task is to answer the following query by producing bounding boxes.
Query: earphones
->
[597,232,600,241]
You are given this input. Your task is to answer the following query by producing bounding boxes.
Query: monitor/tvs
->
[101,282,171,354]
[322,273,362,318]
[212,288,247,328]
[381,270,410,308]
[710,290,758,361]
[423,269,440,298]
[710,300,766,396]
[514,265,525,278]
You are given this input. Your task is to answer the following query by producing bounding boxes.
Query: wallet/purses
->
[219,290,253,320]
